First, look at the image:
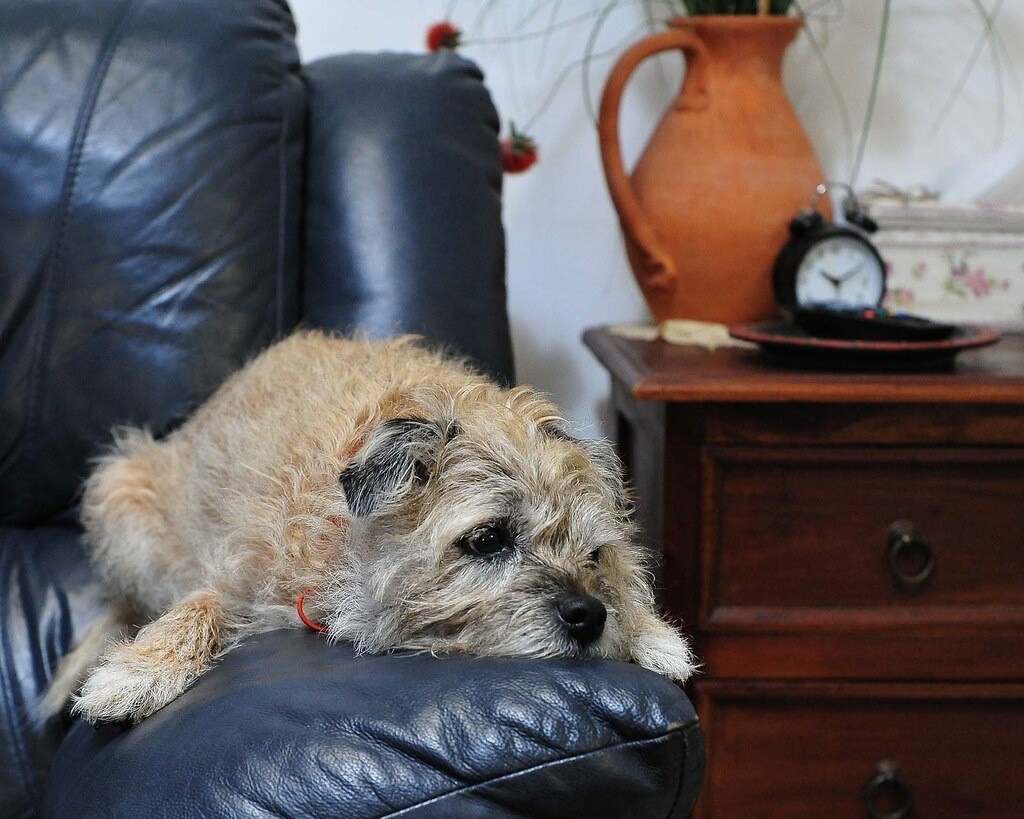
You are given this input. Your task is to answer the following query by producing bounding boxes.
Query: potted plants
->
[442,0,1024,334]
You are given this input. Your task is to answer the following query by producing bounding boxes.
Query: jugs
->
[599,16,833,336]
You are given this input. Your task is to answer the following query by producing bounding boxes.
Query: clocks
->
[771,178,890,319]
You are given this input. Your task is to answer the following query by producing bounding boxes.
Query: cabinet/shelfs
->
[581,334,1024,819]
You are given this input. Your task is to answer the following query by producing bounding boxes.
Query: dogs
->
[33,329,696,728]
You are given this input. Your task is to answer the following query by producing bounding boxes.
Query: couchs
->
[1,0,708,819]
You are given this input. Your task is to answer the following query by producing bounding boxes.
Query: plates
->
[731,312,1002,372]
